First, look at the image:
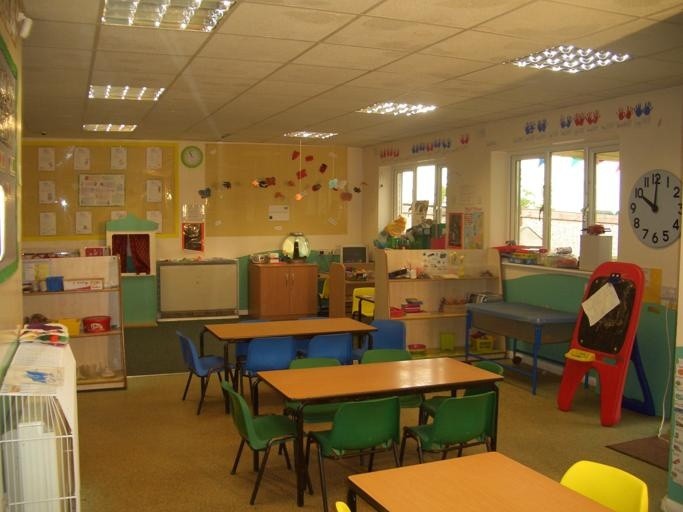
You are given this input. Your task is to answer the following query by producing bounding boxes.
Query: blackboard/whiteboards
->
[578,277,636,355]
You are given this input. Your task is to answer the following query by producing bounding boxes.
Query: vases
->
[578,234,613,273]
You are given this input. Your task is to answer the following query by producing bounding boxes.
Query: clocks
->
[625,167,683,250]
[180,145,203,169]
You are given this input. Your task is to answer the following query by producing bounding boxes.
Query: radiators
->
[1,338,83,511]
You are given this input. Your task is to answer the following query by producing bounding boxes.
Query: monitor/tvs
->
[340,244,368,266]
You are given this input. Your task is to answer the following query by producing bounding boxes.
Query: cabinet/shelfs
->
[20,252,128,393]
[327,262,375,319]
[373,246,508,364]
[245,262,319,322]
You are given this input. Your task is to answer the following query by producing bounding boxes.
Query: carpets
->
[605,430,669,473]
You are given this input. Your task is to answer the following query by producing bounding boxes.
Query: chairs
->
[319,279,329,312]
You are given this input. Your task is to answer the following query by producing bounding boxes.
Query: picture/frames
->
[447,211,465,250]
[77,245,113,259]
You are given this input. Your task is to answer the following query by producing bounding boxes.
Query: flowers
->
[582,224,610,237]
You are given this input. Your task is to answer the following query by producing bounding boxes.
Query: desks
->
[317,272,329,279]
